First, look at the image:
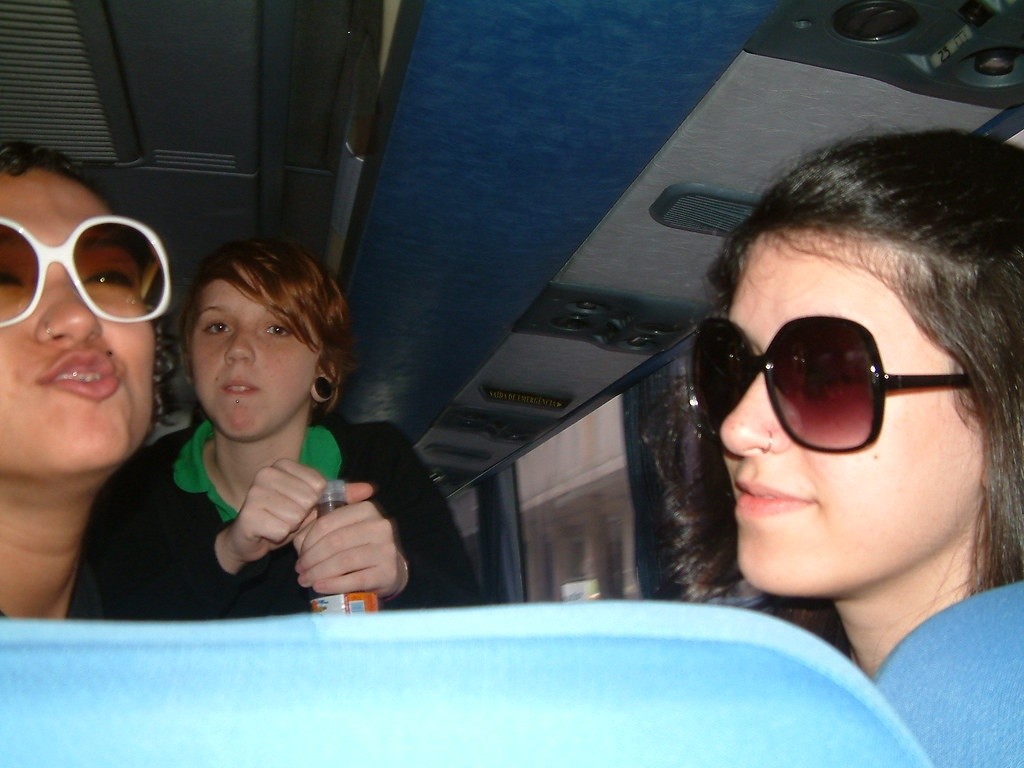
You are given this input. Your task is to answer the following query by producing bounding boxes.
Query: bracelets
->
[377,546,404,601]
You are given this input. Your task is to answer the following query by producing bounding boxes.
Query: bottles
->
[309,479,378,613]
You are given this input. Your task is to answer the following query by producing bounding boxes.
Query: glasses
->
[690,316,975,454]
[0,213,173,330]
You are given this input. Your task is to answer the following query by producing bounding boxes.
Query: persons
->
[86,234,475,619]
[643,126,1024,680]
[0,140,181,618]
